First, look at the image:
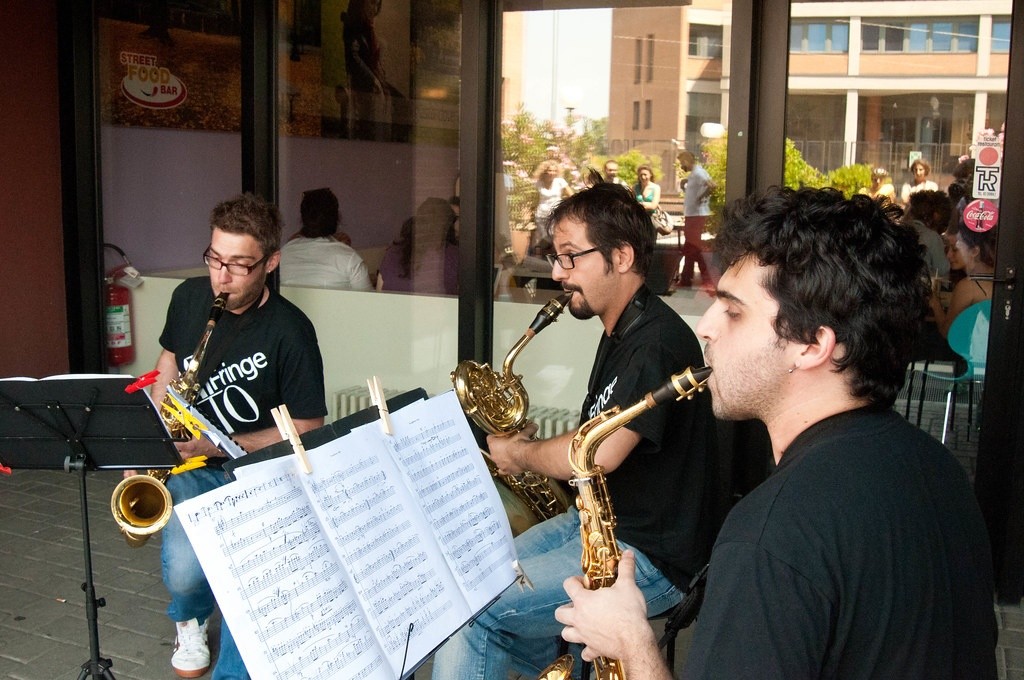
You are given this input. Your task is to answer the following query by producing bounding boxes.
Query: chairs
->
[557,386,770,680]
[904,300,993,435]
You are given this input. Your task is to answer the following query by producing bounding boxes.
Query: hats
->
[297,189,339,238]
[417,196,460,222]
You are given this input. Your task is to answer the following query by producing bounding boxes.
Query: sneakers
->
[171,614,212,677]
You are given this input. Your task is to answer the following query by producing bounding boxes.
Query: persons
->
[125,188,328,680]
[893,188,992,342]
[430,179,736,680]
[524,145,719,293]
[277,185,462,296]
[861,153,974,206]
[555,186,999,680]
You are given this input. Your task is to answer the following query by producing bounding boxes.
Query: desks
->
[673,224,688,282]
[514,268,563,286]
[493,286,572,306]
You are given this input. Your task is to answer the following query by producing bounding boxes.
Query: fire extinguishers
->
[104,263,134,367]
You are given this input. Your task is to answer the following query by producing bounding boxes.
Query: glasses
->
[546,247,601,270]
[202,244,269,275]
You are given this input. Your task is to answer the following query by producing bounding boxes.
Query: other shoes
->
[675,279,692,287]
[706,280,717,296]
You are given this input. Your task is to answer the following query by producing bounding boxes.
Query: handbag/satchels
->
[650,210,674,234]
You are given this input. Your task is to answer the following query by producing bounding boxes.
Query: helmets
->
[872,168,890,180]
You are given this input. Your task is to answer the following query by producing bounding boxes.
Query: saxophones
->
[108,291,230,547]
[533,363,713,680]
[450,289,570,534]
